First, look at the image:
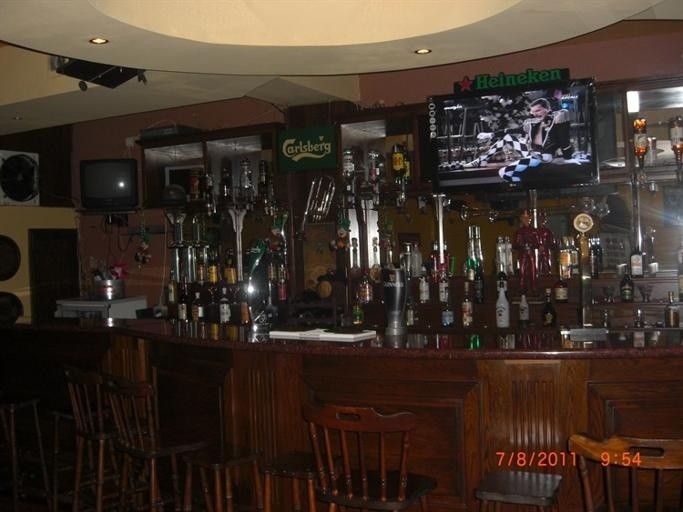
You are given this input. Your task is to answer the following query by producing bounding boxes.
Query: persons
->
[438,97,590,182]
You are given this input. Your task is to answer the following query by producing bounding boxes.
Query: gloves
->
[564,158,590,165]
[476,133,491,140]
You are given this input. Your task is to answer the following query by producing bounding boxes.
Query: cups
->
[238,322,269,344]
[408,334,515,351]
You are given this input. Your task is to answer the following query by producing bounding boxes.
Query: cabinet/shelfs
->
[584,277,683,345]
[137,77,681,331]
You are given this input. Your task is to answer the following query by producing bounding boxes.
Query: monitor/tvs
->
[81,158,137,207]
[425,76,600,192]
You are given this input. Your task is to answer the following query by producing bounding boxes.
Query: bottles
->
[633,118,648,170]
[152,295,168,319]
[167,246,238,324]
[340,143,413,188]
[173,320,239,343]
[668,117,683,168]
[349,223,682,334]
[91,269,104,281]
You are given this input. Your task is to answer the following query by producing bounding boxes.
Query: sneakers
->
[463,157,487,168]
